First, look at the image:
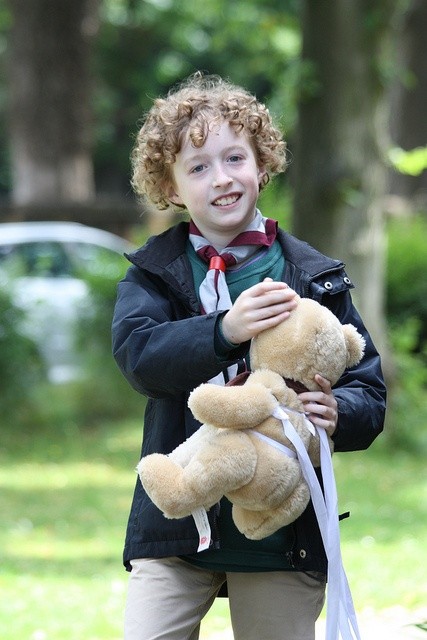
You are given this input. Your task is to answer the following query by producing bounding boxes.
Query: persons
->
[106,72,388,638]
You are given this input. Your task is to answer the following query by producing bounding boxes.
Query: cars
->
[0,220,153,388]
